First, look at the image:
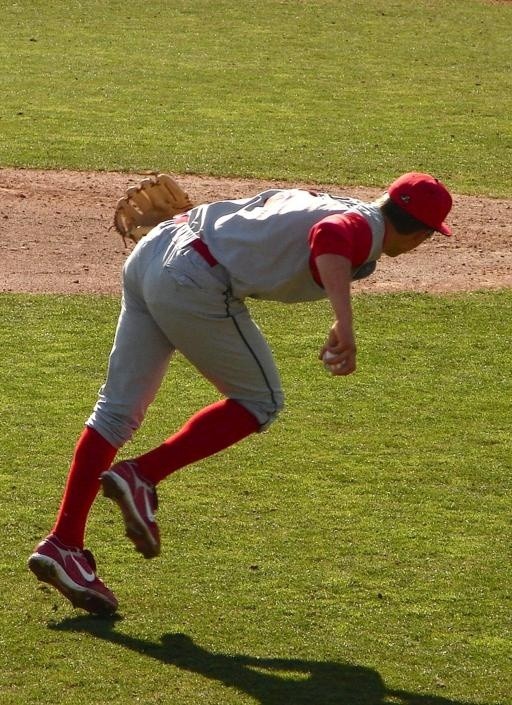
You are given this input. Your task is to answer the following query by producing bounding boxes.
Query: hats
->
[387,172,453,236]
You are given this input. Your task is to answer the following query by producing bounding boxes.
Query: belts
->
[174,214,218,267]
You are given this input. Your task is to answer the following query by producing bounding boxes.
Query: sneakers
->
[101,460,161,558]
[28,535,118,615]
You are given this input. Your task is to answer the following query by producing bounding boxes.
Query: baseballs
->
[322,349,346,371]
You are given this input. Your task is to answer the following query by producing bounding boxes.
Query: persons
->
[26,169,453,616]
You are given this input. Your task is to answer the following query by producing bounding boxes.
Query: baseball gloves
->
[114,174,194,243]
